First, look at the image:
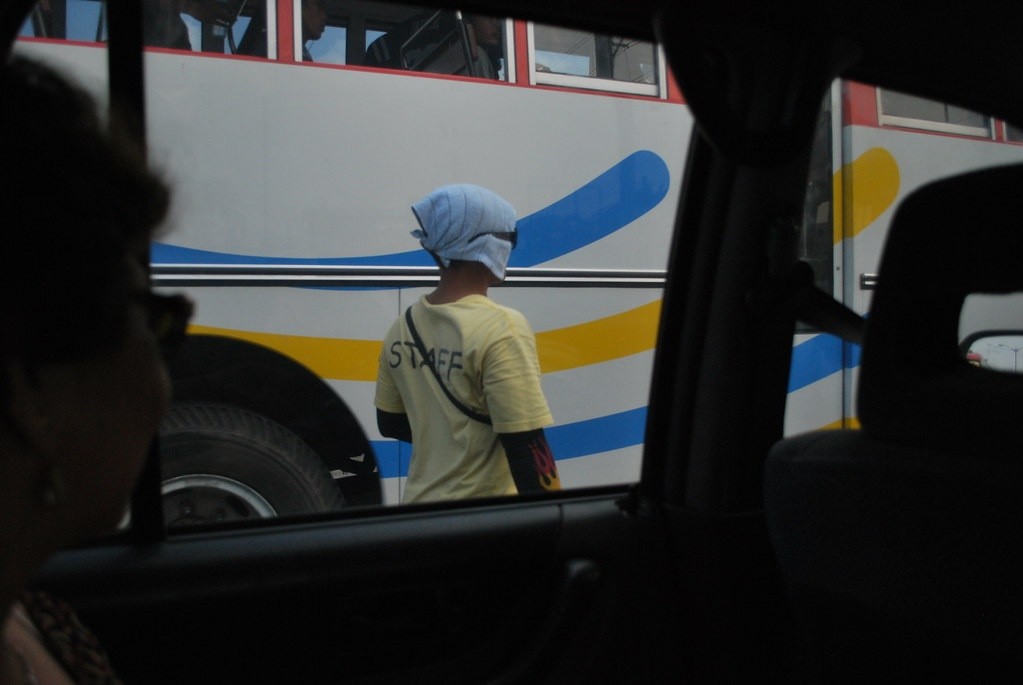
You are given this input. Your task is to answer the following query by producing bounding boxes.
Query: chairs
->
[761,157,1022,685]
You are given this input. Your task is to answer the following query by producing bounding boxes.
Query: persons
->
[375,183,563,505]
[2,47,197,685]
[302,0,330,66]
[458,16,503,82]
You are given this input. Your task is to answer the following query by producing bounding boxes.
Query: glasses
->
[468,227,518,249]
[107,282,194,353]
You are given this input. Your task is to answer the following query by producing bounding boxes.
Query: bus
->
[17,0,1023,529]
